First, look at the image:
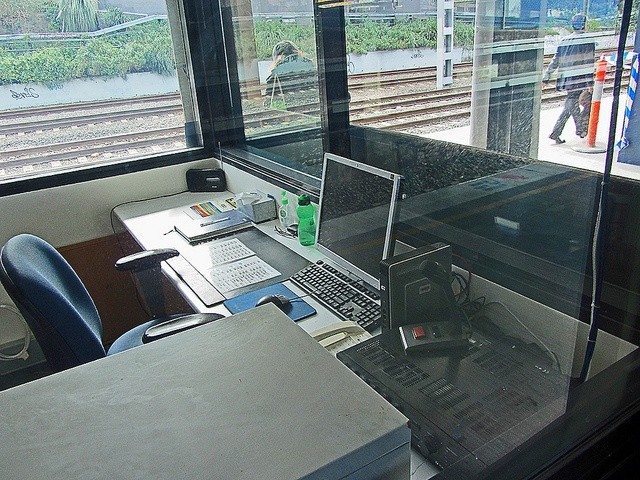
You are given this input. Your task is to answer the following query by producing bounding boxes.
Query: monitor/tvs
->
[314,153,405,291]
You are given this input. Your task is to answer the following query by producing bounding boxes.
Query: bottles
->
[295,193,317,246]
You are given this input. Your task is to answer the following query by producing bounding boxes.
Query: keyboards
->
[289,258,382,332]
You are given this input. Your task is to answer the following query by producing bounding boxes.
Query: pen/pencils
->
[200,215,231,227]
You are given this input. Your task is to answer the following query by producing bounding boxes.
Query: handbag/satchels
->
[264,73,288,125]
[575,91,591,138]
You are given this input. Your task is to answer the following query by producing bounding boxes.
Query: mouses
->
[255,294,293,315]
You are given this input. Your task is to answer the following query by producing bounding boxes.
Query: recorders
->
[186,142,226,192]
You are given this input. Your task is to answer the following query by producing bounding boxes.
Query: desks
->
[113,190,441,480]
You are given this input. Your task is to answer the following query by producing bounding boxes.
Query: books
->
[184,196,242,221]
[173,210,252,242]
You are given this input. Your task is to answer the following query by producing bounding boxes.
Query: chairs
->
[0,231,227,374]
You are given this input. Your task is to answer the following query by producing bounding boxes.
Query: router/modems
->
[379,242,453,336]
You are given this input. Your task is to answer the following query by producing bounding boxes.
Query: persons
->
[541,14,596,144]
[260,40,320,129]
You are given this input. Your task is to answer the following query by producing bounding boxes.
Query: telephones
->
[309,321,374,356]
